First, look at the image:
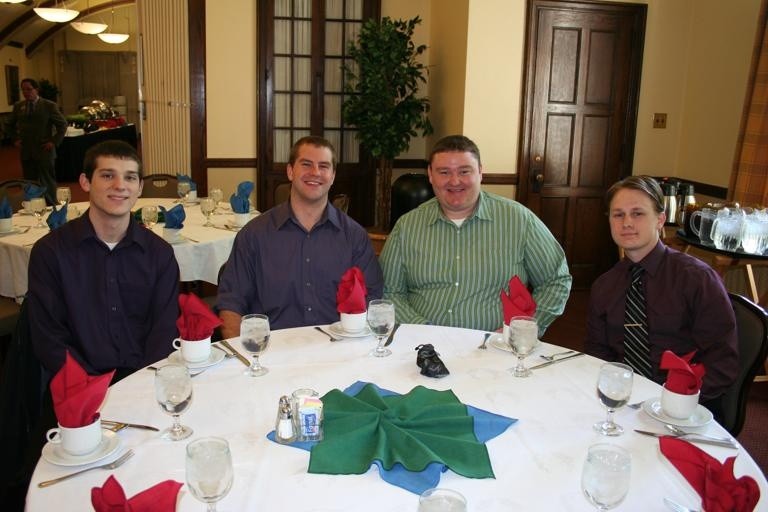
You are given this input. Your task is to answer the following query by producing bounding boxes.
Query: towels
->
[90,473,184,512]
[658,343,708,397]
[655,433,760,512]
[336,268,366,312]
[309,382,495,481]
[500,277,536,323]
[48,346,118,427]
[265,376,519,498]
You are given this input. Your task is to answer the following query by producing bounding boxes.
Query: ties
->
[622,263,654,382]
[28,102,34,120]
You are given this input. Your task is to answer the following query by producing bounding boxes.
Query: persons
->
[587,175,740,429]
[218,136,384,343]
[21,140,181,416]
[379,135,573,345]
[5,78,68,208]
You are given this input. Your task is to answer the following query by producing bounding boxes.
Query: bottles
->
[276,395,299,443]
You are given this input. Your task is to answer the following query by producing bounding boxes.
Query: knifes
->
[635,429,739,450]
[529,353,585,371]
[100,418,160,433]
[221,340,250,366]
[384,322,401,348]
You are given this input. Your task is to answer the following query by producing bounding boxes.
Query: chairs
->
[0,171,351,347]
[700,288,767,436]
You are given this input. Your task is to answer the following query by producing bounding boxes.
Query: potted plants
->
[337,11,439,260]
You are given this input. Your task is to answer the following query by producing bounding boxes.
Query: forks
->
[315,326,343,343]
[212,343,239,359]
[478,332,491,352]
[660,496,697,512]
[35,448,136,490]
[539,351,575,362]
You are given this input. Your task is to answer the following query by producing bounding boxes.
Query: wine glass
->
[155,363,196,442]
[240,313,270,377]
[509,315,539,377]
[582,442,634,510]
[594,360,634,435]
[19,182,224,230]
[366,298,396,358]
[183,436,235,510]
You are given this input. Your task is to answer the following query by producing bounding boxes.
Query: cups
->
[46,416,103,455]
[173,335,211,363]
[160,226,183,243]
[292,387,326,441]
[0,217,12,233]
[501,320,512,345]
[339,309,367,333]
[418,487,468,512]
[232,213,251,228]
[662,382,700,419]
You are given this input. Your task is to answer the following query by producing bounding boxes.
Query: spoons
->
[664,424,735,441]
[101,423,128,433]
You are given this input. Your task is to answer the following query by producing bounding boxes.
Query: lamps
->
[30,0,130,46]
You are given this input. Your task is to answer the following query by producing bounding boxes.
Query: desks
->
[593,195,767,386]
[59,120,138,184]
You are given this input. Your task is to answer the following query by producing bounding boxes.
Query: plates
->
[41,429,119,466]
[168,343,227,368]
[644,395,714,426]
[489,333,516,353]
[328,319,375,337]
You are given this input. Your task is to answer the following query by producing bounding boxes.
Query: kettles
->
[676,199,768,256]
[655,175,697,226]
